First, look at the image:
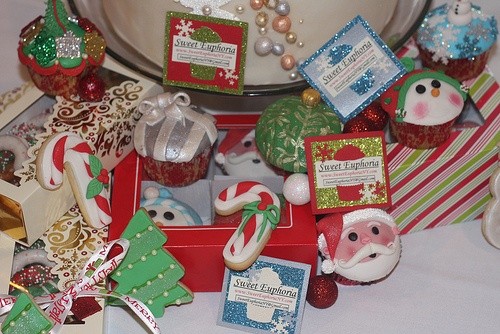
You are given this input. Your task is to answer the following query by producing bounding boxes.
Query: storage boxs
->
[0,56,163,248]
[108,115,319,292]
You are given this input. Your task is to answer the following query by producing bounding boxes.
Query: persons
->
[316,206,402,281]
[215,129,275,177]
